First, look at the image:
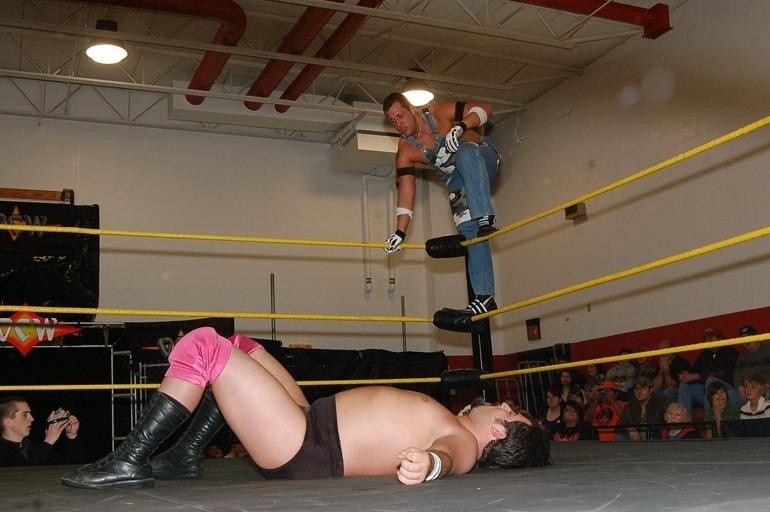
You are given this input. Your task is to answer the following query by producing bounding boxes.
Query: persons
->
[0,394,90,468]
[378,92,502,314]
[60,326,556,488]
[497,326,770,443]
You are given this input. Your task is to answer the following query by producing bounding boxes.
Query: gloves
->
[383,229,406,256]
[445,122,467,153]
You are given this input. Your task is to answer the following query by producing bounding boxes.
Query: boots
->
[60,389,192,490]
[151,388,226,481]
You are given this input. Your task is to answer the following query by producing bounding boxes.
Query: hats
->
[596,380,619,391]
[739,325,758,335]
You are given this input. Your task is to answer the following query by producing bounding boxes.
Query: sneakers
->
[463,294,497,315]
[476,215,499,242]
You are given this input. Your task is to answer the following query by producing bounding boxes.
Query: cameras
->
[50,407,81,421]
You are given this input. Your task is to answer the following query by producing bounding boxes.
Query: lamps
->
[83,16,131,69]
[397,64,438,110]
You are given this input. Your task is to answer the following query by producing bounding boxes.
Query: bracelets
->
[395,206,413,219]
[467,106,488,128]
[425,452,442,481]
